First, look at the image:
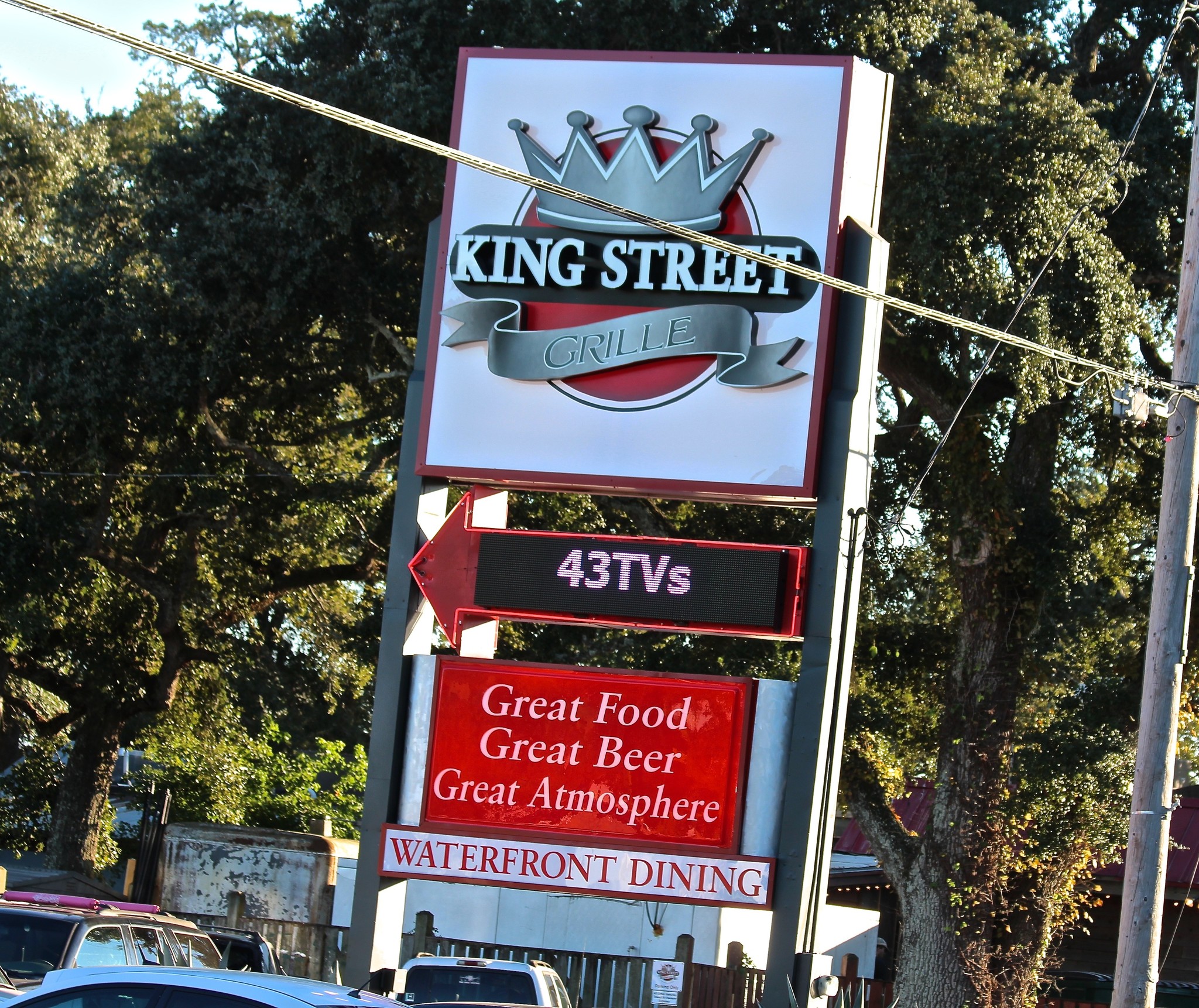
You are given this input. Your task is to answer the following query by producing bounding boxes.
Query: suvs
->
[194,922,283,974]
[0,893,229,992]
[394,952,573,1008]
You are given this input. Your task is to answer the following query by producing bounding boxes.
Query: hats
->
[876,938,890,952]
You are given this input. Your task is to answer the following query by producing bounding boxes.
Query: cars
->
[0,964,414,1008]
